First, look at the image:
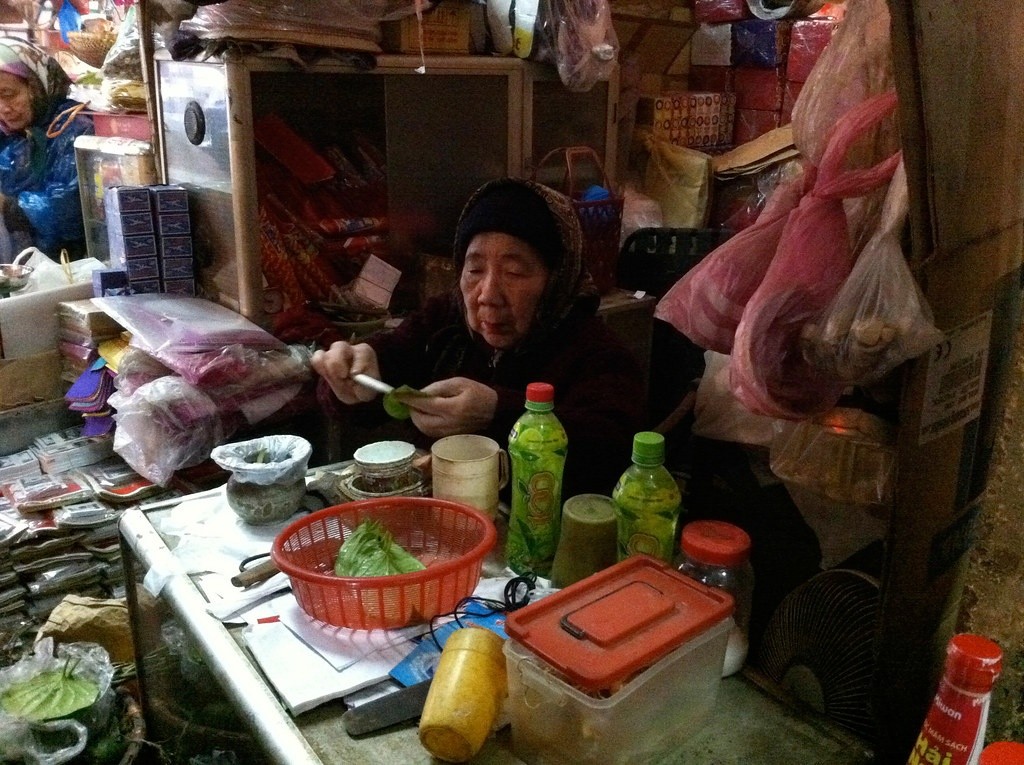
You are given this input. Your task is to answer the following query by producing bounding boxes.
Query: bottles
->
[613,433,680,569]
[906,634,1005,764]
[506,380,569,577]
[675,519,752,680]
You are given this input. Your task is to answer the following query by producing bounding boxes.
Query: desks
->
[119,464,874,765]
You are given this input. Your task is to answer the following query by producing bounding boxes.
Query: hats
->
[454,181,556,269]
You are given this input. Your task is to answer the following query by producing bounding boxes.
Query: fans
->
[760,568,922,755]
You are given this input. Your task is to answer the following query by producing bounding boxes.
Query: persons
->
[310,175,649,510]
[27,0,89,54]
[0,37,95,263]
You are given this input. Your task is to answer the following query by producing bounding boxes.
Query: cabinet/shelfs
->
[151,50,616,345]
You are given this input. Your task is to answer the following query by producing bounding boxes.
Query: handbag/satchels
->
[45,72,137,138]
[652,0,944,423]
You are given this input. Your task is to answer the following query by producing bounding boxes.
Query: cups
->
[353,440,416,493]
[417,627,508,761]
[550,495,618,589]
[431,433,507,522]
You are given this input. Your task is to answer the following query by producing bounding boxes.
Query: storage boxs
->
[390,0,1022,664]
[93,184,196,299]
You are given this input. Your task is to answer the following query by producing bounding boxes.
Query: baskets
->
[530,146,625,296]
[65,30,118,69]
[115,685,148,765]
[269,496,498,630]
[149,695,265,764]
